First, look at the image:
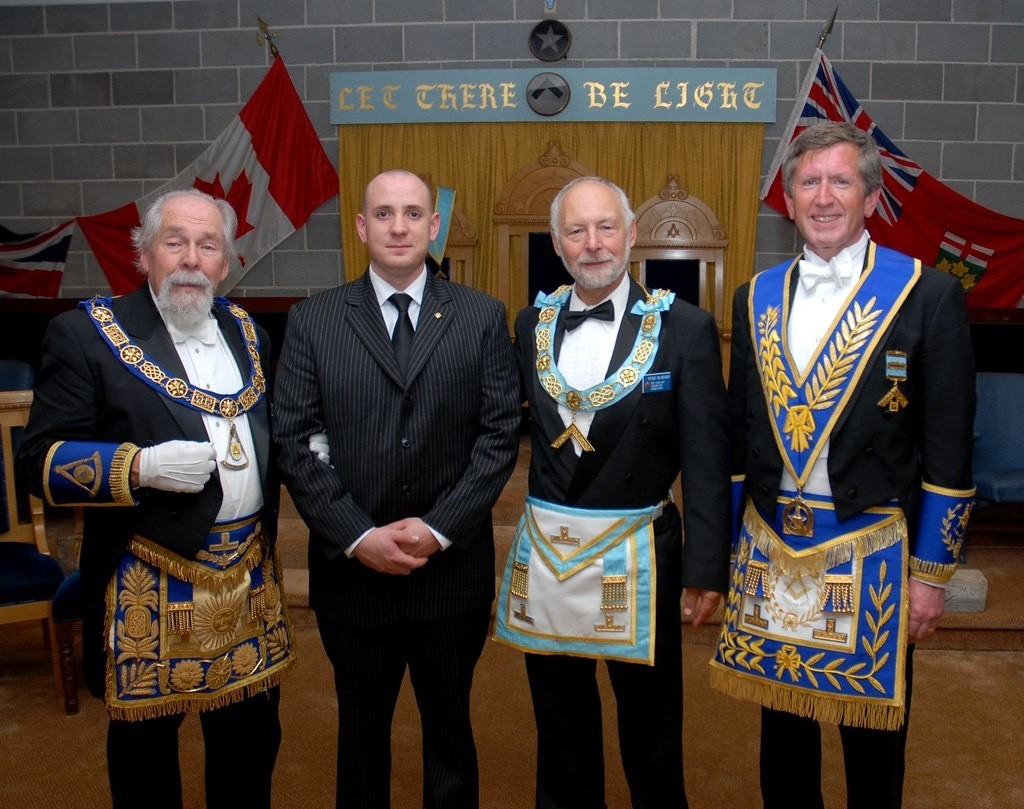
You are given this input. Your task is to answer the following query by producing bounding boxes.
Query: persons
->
[15,190,329,809]
[273,169,523,809]
[727,122,977,809]
[511,176,730,809]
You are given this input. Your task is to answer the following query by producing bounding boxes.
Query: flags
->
[76,55,338,300]
[760,46,1024,325]
[0,222,75,300]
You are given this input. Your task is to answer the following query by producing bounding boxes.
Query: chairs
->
[0,390,68,697]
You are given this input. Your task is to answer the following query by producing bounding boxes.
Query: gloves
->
[139,440,216,493]
[309,429,334,469]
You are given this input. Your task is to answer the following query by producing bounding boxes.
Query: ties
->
[388,294,415,381]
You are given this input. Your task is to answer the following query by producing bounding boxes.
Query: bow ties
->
[561,300,614,333]
[799,252,852,291]
[168,320,217,344]
